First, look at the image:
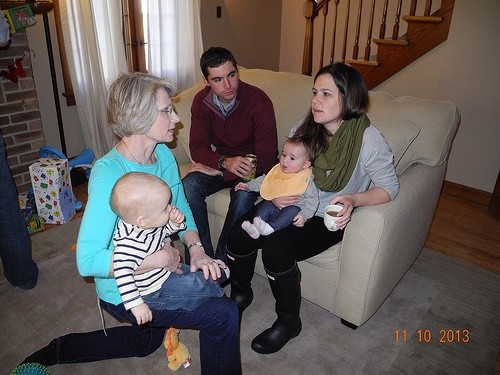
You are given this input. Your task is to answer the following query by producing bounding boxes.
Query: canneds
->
[242,153,257,180]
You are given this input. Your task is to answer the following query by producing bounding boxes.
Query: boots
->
[225,248,256,313]
[250,261,303,354]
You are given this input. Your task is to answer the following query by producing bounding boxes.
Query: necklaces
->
[122,141,145,166]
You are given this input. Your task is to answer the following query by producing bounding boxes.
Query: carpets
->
[0,216,500,375]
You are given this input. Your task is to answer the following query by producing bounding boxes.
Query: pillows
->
[167,82,206,164]
[366,113,421,167]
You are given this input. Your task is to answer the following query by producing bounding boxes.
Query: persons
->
[110,172,225,323]
[234,133,321,239]
[19,72,244,375]
[180,48,282,288]
[223,63,400,355]
[0,136,39,291]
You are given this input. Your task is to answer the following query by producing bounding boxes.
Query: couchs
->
[167,66,461,331]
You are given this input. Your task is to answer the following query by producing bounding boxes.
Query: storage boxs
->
[29,158,76,224]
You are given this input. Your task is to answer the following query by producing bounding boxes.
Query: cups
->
[324,205,343,231]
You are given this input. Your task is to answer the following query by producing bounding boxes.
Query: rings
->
[349,217,352,221]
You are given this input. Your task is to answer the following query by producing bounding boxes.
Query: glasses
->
[153,104,175,115]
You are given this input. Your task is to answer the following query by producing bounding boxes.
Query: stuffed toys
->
[164,327,191,371]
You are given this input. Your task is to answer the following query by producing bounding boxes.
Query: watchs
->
[189,241,203,249]
[220,156,227,169]
